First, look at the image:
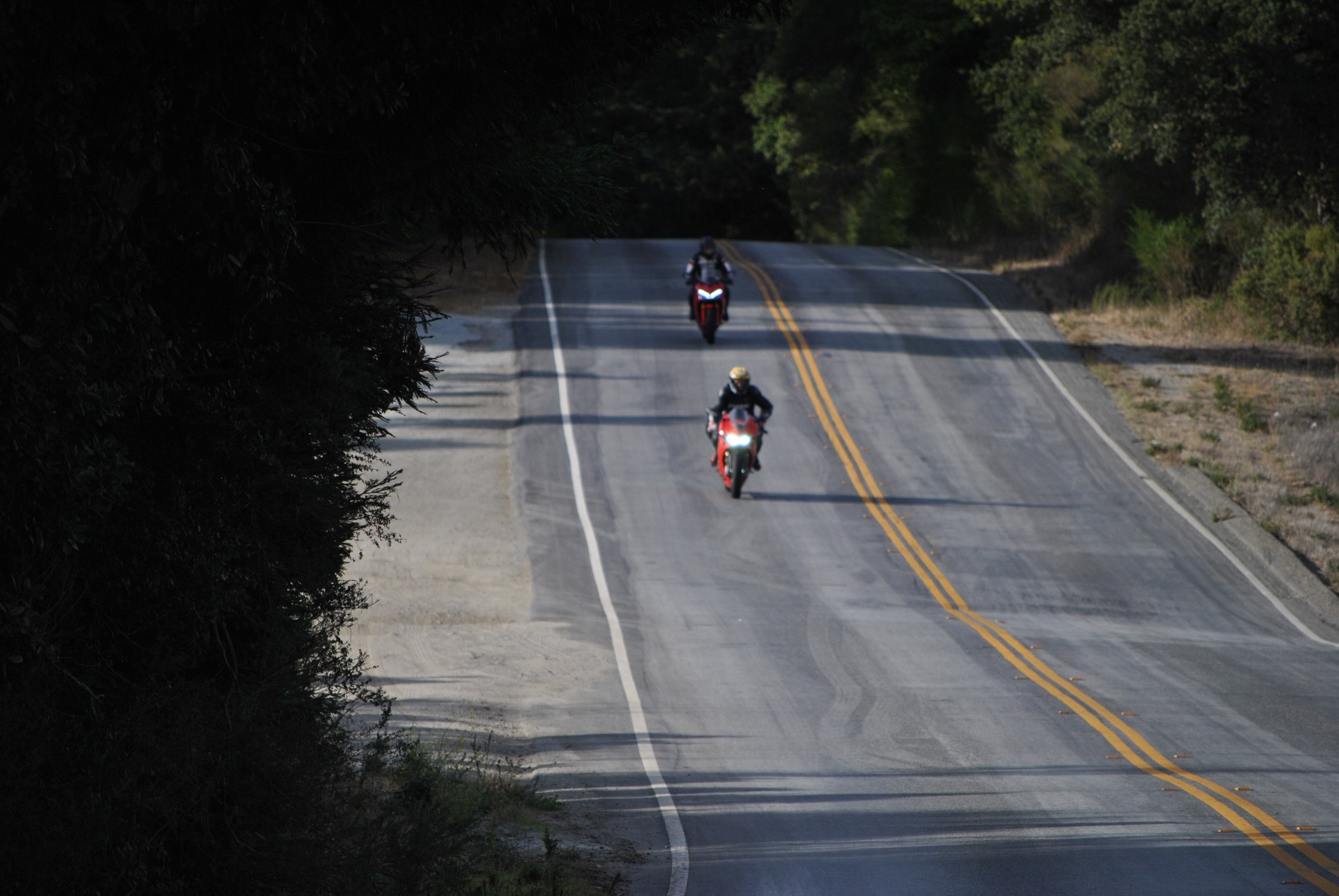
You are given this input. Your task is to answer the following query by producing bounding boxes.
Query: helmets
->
[728,366,749,395]
[700,237,717,260]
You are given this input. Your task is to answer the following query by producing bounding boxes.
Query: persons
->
[684,236,733,321]
[704,367,773,471]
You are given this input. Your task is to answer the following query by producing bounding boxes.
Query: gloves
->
[707,421,717,432]
[727,277,736,285]
[686,279,694,286]
[757,419,764,426]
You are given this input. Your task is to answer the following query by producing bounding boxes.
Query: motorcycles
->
[684,258,734,342]
[714,407,759,499]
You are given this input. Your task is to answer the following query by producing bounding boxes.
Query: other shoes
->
[753,456,761,471]
[689,310,696,320]
[723,312,729,321]
[710,451,717,466]
